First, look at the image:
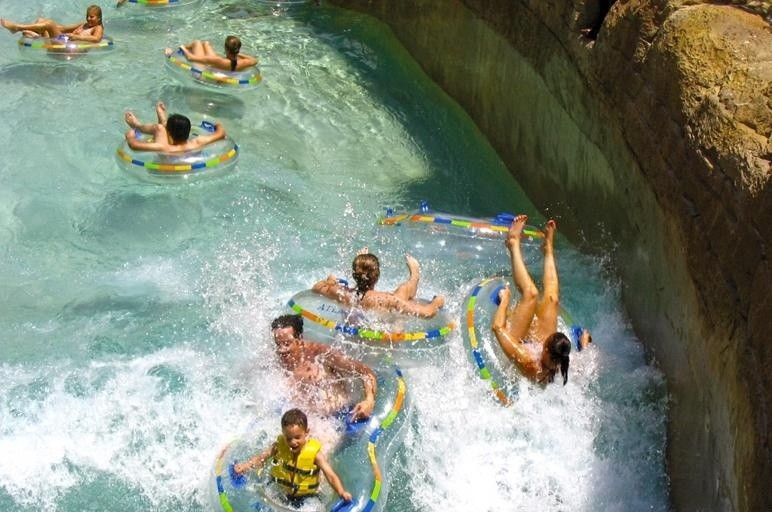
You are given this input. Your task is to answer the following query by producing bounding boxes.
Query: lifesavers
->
[375,202,547,264]
[460,276,586,408]
[16,34,115,61]
[283,280,454,366]
[164,48,262,90]
[107,0,198,14]
[208,354,412,511]
[115,120,240,185]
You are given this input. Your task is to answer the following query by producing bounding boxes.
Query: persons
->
[312,244,448,330]
[164,35,259,80]
[241,314,378,463]
[232,408,353,511]
[1,5,105,61]
[120,101,228,158]
[492,211,594,390]
